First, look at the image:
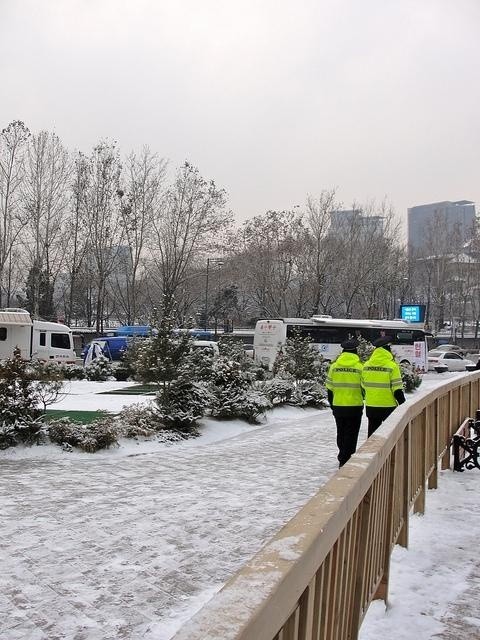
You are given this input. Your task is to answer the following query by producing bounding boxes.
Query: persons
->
[360,336,406,438]
[326,339,366,470]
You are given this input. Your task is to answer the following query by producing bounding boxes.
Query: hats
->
[340,338,359,350]
[372,336,393,347]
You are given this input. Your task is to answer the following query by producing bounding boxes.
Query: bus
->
[253,315,434,372]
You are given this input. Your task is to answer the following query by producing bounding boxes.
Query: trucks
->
[0,308,219,364]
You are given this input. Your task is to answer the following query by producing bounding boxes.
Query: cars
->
[428,339,480,373]
